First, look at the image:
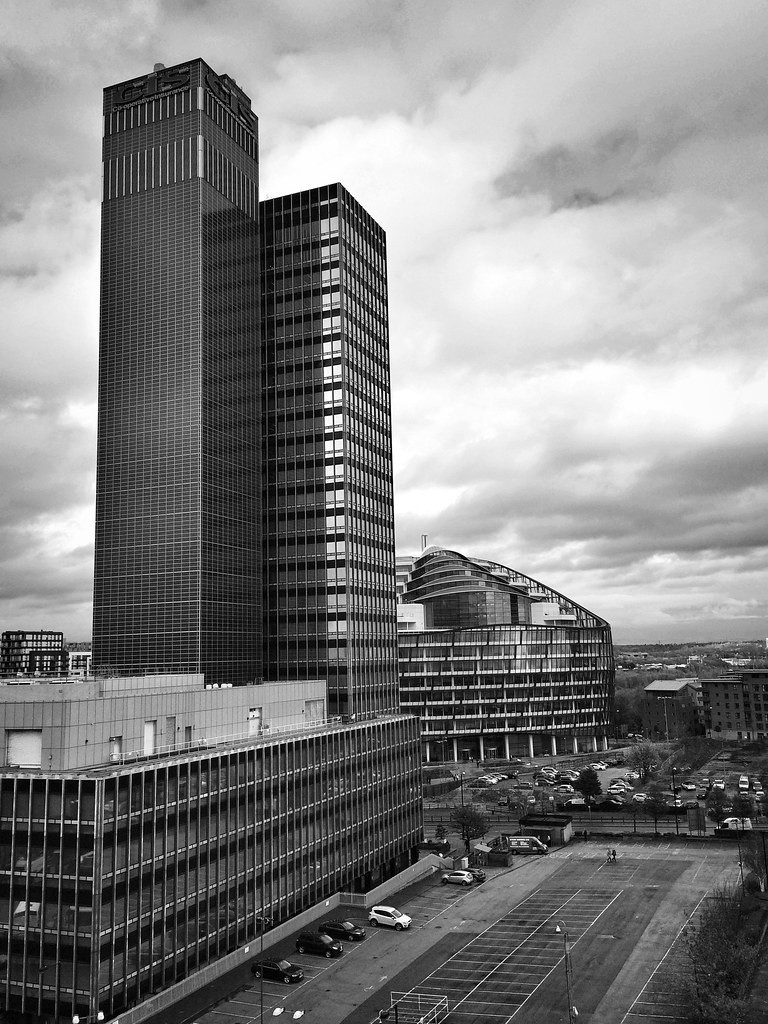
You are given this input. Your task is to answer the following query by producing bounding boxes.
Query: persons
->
[607,848,616,863]
[582,828,588,841]
[477,759,479,768]
[464,837,471,853]
[453,774,459,781]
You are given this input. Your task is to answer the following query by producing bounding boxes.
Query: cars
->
[319,919,366,941]
[251,958,304,984]
[476,760,765,812]
[461,868,486,883]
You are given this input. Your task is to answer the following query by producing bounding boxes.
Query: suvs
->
[368,906,413,931]
[441,870,473,886]
[296,931,344,958]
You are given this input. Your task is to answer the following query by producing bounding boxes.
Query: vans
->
[508,836,549,855]
[716,817,752,830]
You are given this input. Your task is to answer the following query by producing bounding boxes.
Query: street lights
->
[556,920,573,1024]
[461,771,466,840]
[442,737,447,766]
[673,767,680,837]
[255,916,274,1024]
